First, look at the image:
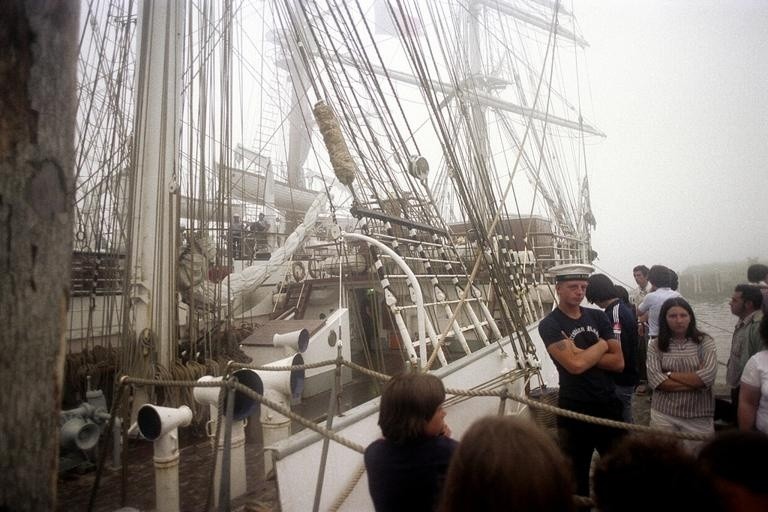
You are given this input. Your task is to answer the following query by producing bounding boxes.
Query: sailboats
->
[57,1,601,512]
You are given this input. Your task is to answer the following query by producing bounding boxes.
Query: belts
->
[647,335,656,339]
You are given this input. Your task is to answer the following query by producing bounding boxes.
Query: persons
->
[538,263,768,512]
[365,372,459,512]
[257,214,271,231]
[232,214,244,258]
[439,415,572,512]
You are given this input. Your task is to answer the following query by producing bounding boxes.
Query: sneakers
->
[635,384,650,396]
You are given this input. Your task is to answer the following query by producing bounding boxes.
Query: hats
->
[547,264,595,281]
